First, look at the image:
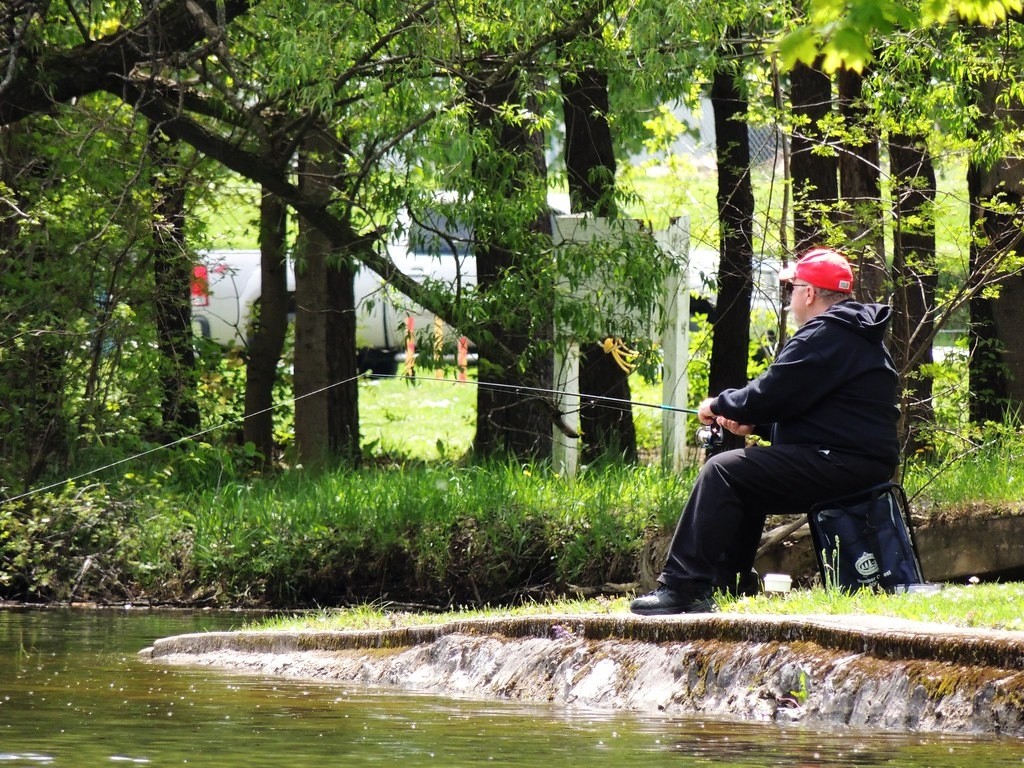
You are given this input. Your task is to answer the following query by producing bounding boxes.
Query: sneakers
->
[630,583,714,615]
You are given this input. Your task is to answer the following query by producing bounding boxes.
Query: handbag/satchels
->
[818,490,917,596]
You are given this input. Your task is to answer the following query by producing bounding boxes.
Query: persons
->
[630,249,902,616]
[752,330,778,368]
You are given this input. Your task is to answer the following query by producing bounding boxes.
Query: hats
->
[779,249,853,294]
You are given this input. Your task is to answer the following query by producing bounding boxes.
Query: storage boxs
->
[764,573,792,592]
[894,583,942,597]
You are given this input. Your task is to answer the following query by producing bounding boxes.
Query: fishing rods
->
[367,370,720,422]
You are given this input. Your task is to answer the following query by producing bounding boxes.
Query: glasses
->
[785,282,809,294]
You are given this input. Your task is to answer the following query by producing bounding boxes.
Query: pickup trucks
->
[189,188,794,350]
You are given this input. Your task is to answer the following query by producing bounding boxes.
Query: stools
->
[807,482,926,591]
